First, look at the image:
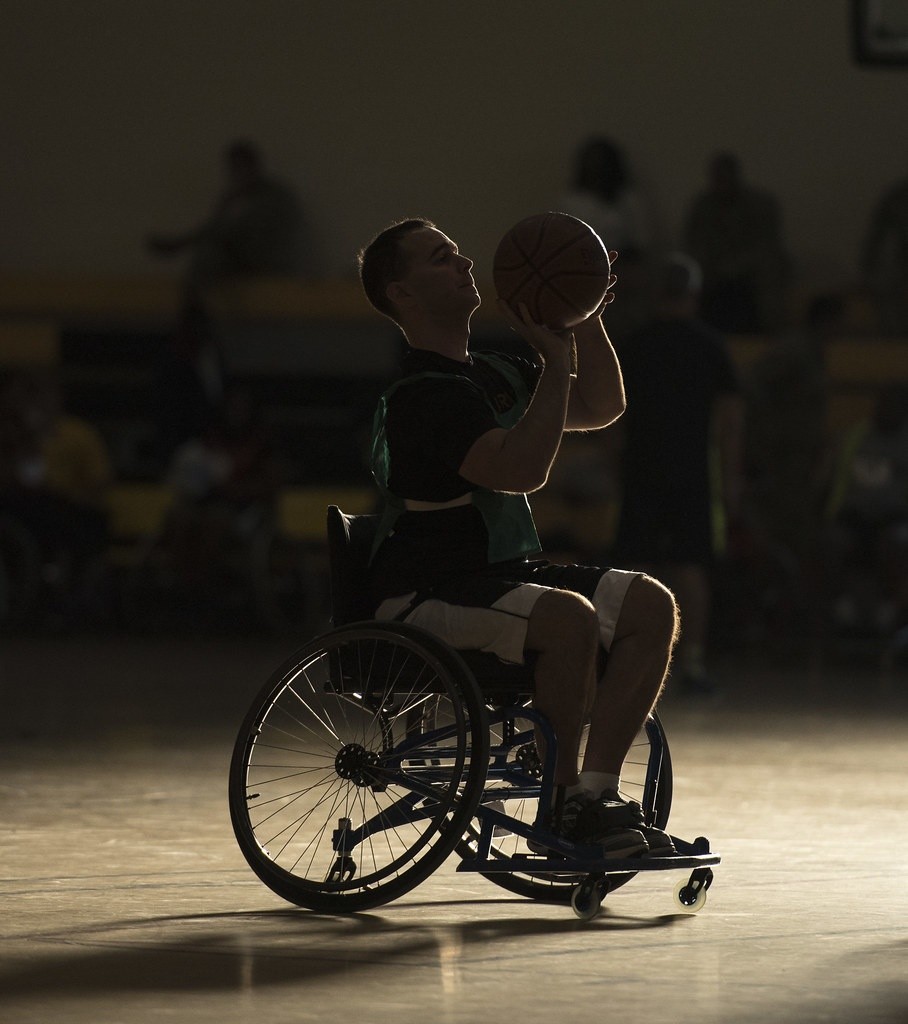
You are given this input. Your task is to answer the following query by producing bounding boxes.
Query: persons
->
[0,133,320,638]
[611,256,743,692]
[818,278,908,670]
[359,219,677,858]
[560,134,660,250]
[684,146,791,338]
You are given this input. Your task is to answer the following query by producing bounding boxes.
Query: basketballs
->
[491,209,613,332]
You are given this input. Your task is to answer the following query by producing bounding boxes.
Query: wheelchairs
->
[227,504,719,919]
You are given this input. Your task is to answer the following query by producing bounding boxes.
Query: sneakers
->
[600,788,677,854]
[532,792,650,860]
